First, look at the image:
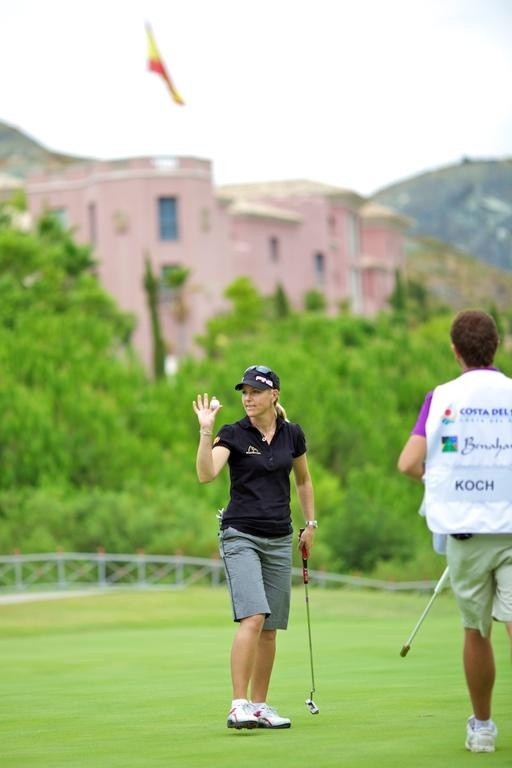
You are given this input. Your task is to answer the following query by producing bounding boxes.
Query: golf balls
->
[210,399,219,409]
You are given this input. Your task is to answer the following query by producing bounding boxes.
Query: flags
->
[139,19,189,111]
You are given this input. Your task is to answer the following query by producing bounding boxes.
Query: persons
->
[190,362,317,730]
[393,306,511,756]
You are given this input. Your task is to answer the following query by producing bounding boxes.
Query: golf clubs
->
[301,528,321,714]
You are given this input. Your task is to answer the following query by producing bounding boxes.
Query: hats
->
[233,362,287,393]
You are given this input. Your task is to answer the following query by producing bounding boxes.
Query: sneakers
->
[250,701,290,731]
[461,714,498,754]
[227,698,259,730]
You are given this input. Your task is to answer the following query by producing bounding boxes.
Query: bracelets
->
[305,520,317,528]
[200,429,212,436]
